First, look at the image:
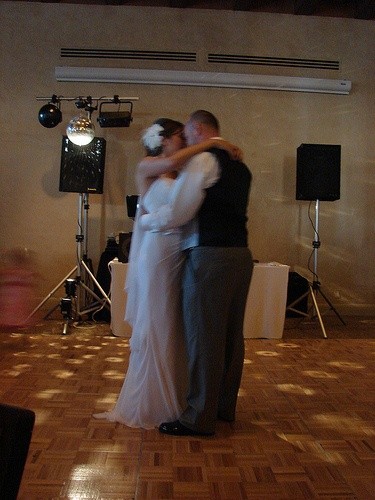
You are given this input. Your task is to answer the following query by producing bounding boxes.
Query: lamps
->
[61,298,72,316]
[65,278,76,295]
[97,99,133,128]
[38,99,62,128]
[66,113,95,145]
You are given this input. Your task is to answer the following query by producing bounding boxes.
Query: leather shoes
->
[159,419,199,436]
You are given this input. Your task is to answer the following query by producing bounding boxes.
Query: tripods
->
[287,201,346,339]
[22,191,114,334]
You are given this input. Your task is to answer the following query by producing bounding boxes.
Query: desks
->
[112,261,289,339]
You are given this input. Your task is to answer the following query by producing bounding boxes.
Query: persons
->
[92,118,245,430]
[139,109,254,437]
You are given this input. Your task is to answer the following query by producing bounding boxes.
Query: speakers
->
[58,136,105,193]
[295,144,341,201]
